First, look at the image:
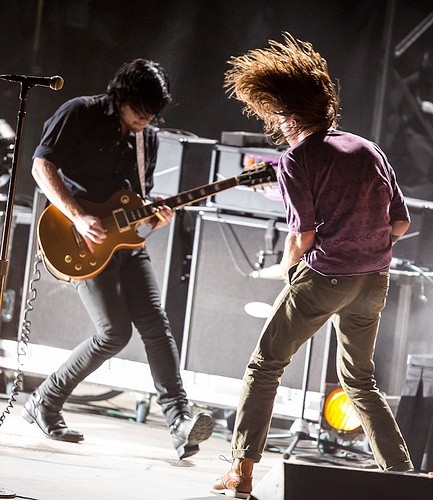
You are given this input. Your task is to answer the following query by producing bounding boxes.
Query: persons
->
[208,31,414,500]
[19,58,214,461]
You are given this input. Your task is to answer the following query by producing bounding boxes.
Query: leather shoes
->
[211,457,254,499]
[21,393,84,441]
[169,412,214,459]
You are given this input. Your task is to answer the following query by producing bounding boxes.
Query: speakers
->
[16,132,432,422]
[284,462,433,500]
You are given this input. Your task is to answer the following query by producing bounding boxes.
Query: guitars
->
[37,161,278,282]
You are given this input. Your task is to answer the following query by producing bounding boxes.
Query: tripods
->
[265,334,375,460]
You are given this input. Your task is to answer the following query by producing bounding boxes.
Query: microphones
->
[0,74,64,90]
